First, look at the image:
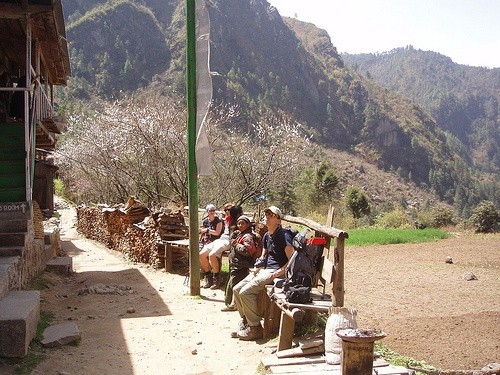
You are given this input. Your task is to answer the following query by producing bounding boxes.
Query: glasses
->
[209,210,215,212]
[224,209,230,212]
[266,212,276,217]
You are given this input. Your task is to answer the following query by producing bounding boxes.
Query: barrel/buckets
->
[342,340,373,375]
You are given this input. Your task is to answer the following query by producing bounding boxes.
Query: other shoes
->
[231,321,263,340]
[221,304,237,311]
[203,274,213,288]
[210,271,223,289]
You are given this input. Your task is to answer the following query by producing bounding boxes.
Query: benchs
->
[247,213,349,351]
[164,238,231,272]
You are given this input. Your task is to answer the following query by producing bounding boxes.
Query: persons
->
[198,202,295,341]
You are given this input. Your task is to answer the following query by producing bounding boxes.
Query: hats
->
[237,215,251,224]
[264,206,282,218]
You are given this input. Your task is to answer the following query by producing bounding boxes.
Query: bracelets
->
[273,271,280,278]
[205,228,209,234]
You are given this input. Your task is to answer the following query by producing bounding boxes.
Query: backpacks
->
[238,232,263,258]
[204,216,225,238]
[264,226,300,270]
[285,237,327,288]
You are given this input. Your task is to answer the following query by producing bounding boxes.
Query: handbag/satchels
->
[285,285,313,303]
